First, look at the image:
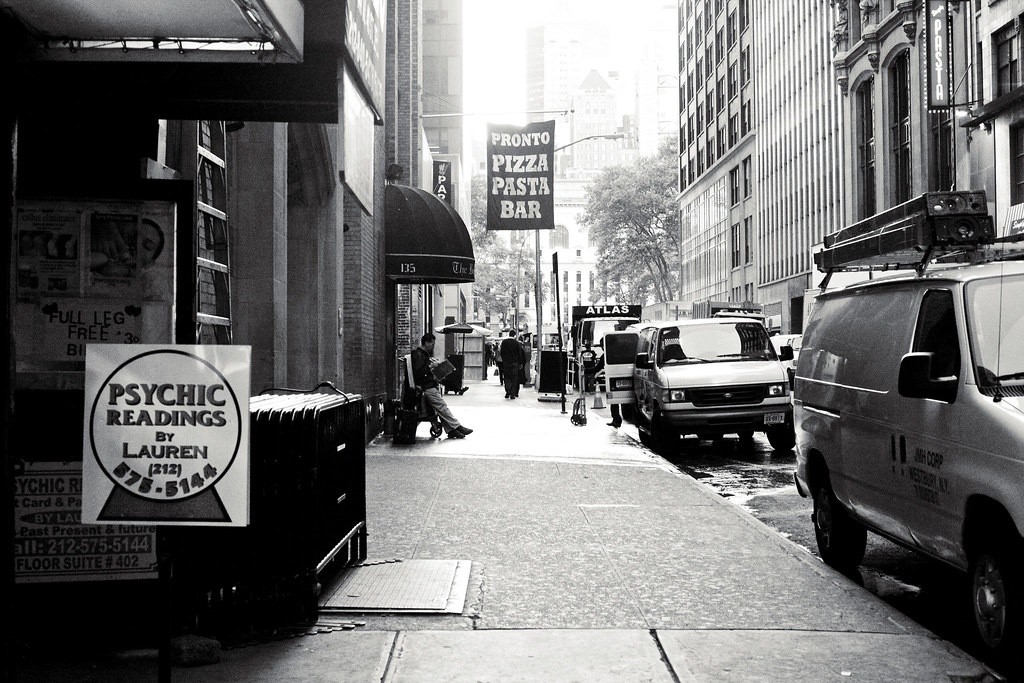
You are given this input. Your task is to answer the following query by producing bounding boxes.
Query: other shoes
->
[448,429,465,439]
[511,394,515,399]
[606,422,620,427]
[457,425,473,435]
[516,393,518,397]
[505,392,510,399]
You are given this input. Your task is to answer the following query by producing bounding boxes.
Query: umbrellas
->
[434,321,493,354]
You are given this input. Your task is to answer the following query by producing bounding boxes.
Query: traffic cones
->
[590,381,606,409]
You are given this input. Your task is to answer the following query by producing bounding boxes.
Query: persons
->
[137,218,170,301]
[484,339,528,385]
[500,329,521,399]
[411,334,473,439]
[587,337,631,428]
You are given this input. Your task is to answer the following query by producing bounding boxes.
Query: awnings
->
[384,184,477,284]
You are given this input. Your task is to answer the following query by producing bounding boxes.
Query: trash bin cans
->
[539,350,567,394]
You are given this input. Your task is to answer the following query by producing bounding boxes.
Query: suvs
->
[513,314,805,393]
[787,186,1024,670]
[603,306,796,456]
